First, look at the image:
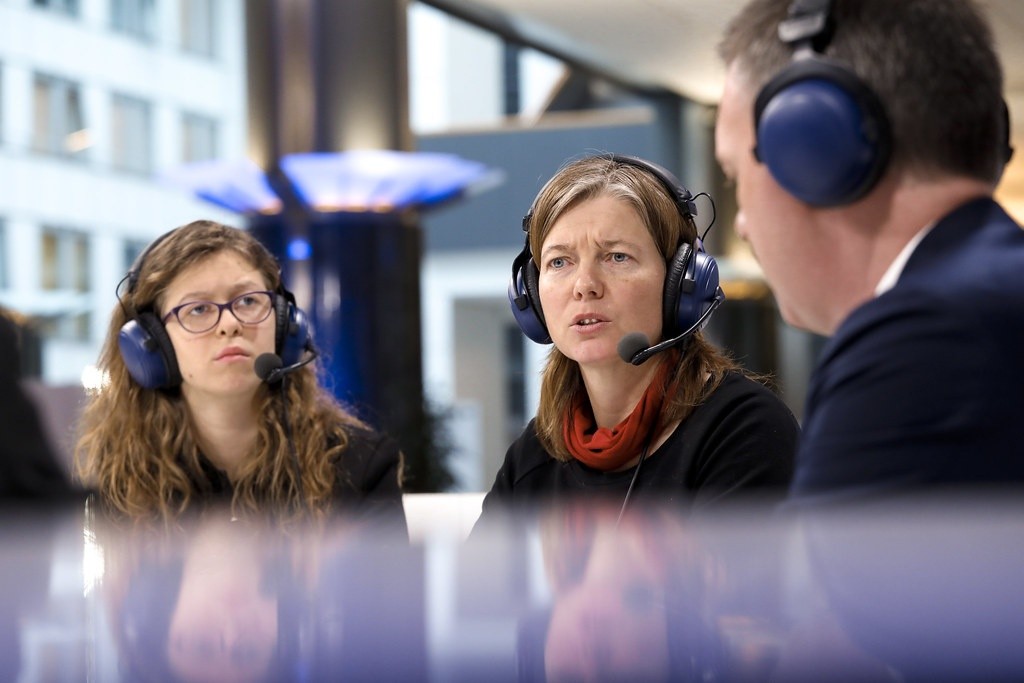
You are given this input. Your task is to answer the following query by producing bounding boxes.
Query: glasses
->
[163,290,276,333]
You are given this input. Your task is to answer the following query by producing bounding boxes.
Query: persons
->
[483,152,797,510]
[468,509,790,683]
[72,220,404,508]
[94,510,411,683]
[0,306,81,512]
[713,0,1024,503]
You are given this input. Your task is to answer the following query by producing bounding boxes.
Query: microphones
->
[254,335,319,383]
[617,286,726,366]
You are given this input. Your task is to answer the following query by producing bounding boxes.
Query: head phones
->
[750,0,896,208]
[116,226,309,397]
[508,153,726,341]
